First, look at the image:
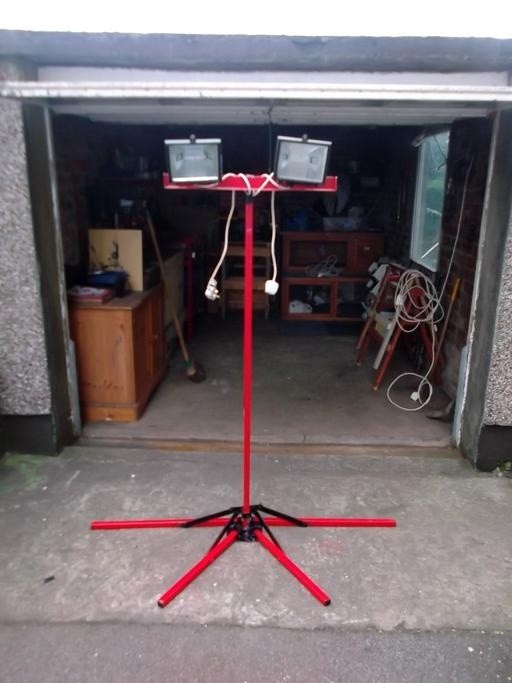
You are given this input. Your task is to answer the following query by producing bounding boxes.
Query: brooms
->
[142,198,205,382]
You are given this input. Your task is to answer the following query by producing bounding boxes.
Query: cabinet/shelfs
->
[218,239,271,320]
[146,228,206,349]
[69,278,164,421]
[278,228,394,329]
